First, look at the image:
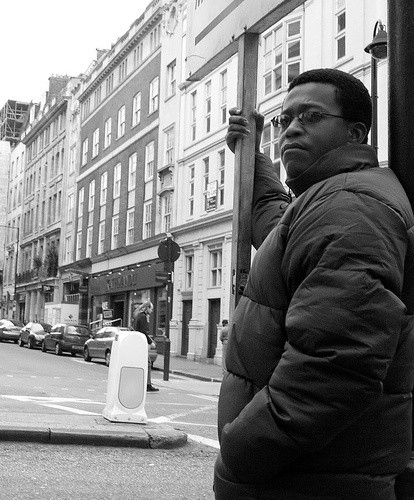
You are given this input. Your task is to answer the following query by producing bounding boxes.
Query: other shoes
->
[147,386,159,391]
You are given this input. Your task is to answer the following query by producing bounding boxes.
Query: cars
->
[18,322,53,349]
[0,319,22,344]
[83,326,158,370]
[42,323,93,358]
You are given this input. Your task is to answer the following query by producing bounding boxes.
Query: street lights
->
[364,19,387,157]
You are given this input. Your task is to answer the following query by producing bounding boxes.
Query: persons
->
[220,320,229,373]
[212,68,414,500]
[132,301,159,392]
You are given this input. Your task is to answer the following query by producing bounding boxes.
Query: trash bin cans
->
[103,331,149,424]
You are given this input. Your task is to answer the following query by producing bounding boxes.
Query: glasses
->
[271,111,352,124]
